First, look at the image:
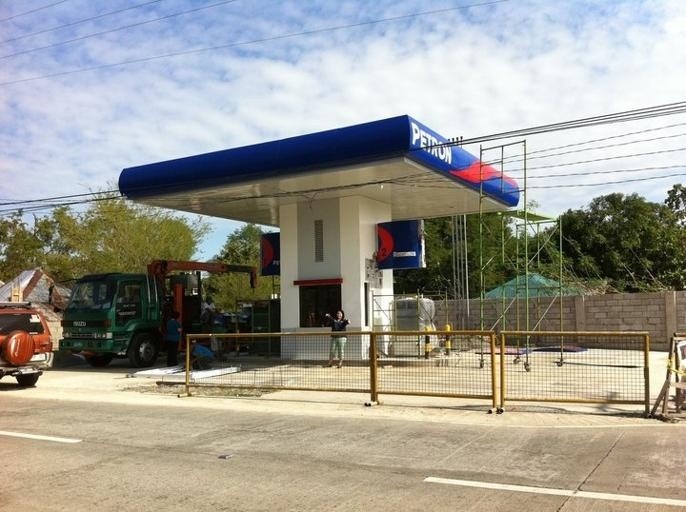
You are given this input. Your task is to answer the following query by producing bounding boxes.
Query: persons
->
[164,311,183,367]
[322,310,351,368]
[199,295,222,327]
[181,341,216,371]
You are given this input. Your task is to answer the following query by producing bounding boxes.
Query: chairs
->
[323,364,343,368]
[168,360,215,371]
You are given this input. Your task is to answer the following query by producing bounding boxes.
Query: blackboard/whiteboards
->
[59,259,257,368]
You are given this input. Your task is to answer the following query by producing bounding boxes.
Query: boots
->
[0,302,52,386]
